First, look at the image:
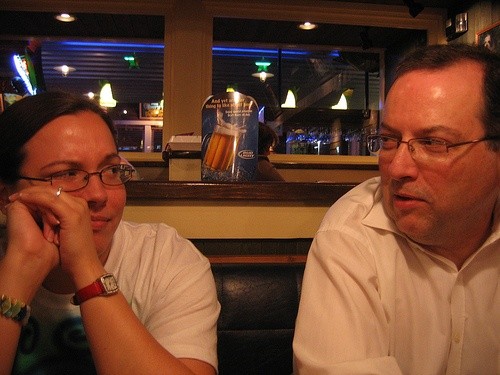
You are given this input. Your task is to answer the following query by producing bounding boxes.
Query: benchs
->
[201,254,305,375]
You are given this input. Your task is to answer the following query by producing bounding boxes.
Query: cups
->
[202,123,244,182]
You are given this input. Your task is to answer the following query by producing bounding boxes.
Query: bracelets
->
[0,295,31,327]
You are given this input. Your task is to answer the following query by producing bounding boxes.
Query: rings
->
[56,187,63,197]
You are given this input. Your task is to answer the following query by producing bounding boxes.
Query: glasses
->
[367,134,500,165]
[19,155,136,193]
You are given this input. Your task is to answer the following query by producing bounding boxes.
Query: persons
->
[257,121,285,182]
[292,44,500,375]
[0,91,222,375]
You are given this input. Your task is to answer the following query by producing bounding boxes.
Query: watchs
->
[70,273,119,306]
